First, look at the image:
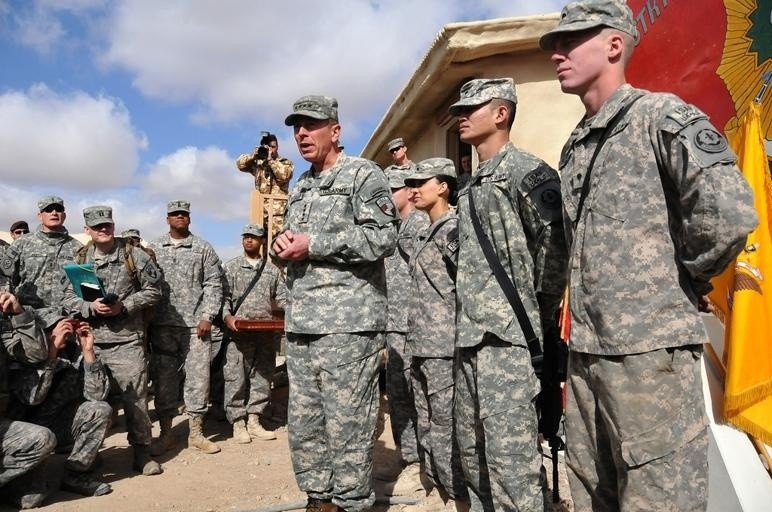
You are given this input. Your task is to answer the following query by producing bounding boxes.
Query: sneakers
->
[372,460,411,483]
[442,496,472,512]
[383,461,436,499]
[13,480,62,510]
[59,472,113,498]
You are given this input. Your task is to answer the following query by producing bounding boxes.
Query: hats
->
[32,306,68,330]
[10,220,29,233]
[239,221,266,238]
[260,134,278,146]
[82,205,114,228]
[166,199,192,214]
[284,94,340,127]
[447,76,519,117]
[538,0,640,52]
[387,137,406,153]
[385,169,413,190]
[403,157,458,188]
[120,227,145,242]
[37,194,65,213]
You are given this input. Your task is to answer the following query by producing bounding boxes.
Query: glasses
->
[14,230,31,235]
[42,204,65,213]
[390,147,402,154]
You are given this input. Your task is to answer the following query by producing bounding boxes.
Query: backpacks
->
[76,239,162,327]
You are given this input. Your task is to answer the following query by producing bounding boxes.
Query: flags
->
[707,98,772,446]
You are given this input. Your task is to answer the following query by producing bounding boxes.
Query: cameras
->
[257,146,269,155]
[68,319,80,329]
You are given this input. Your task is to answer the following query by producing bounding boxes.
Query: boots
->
[231,417,252,445]
[131,444,164,478]
[186,415,223,454]
[246,412,278,443]
[303,497,339,512]
[149,428,179,457]
